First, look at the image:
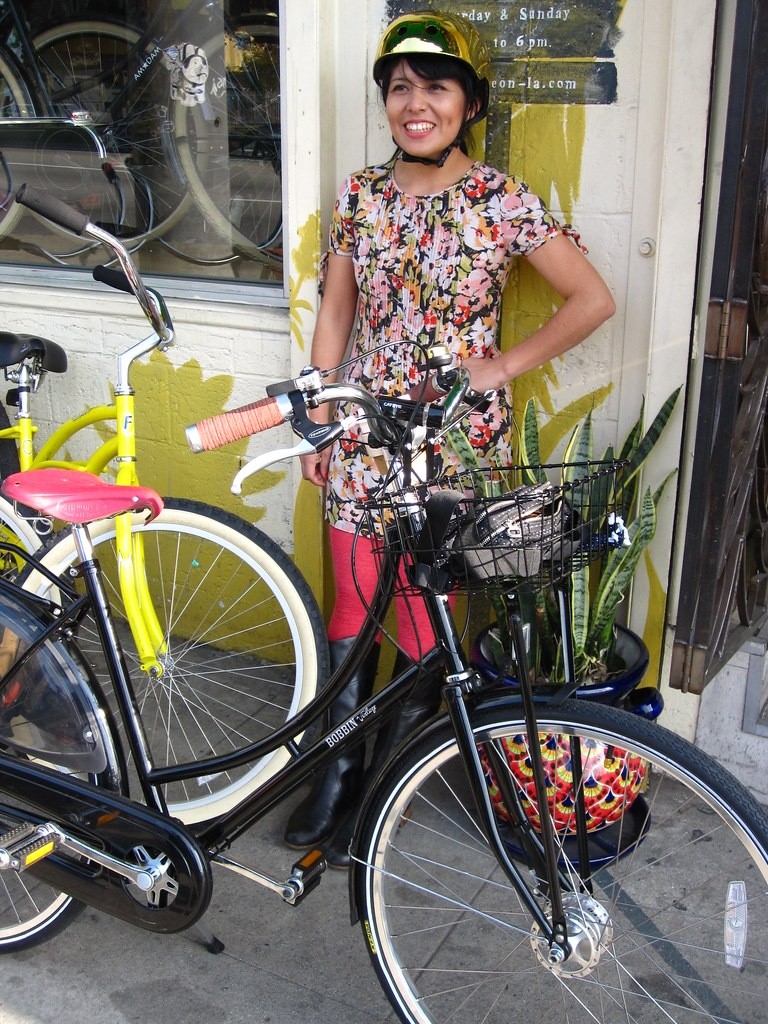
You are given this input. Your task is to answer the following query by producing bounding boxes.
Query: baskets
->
[355,458,631,597]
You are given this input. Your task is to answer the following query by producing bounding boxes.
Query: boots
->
[284,637,381,850]
[326,649,446,871]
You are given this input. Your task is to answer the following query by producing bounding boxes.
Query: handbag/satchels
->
[410,479,571,593]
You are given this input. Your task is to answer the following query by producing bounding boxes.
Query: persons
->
[283,16,616,870]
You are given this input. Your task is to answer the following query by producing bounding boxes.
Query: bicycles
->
[0,1,281,270]
[0,337,768,1023]
[0,183,329,826]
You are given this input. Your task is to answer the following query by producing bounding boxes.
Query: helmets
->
[372,10,490,125]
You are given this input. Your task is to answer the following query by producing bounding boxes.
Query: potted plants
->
[441,384,686,873]
